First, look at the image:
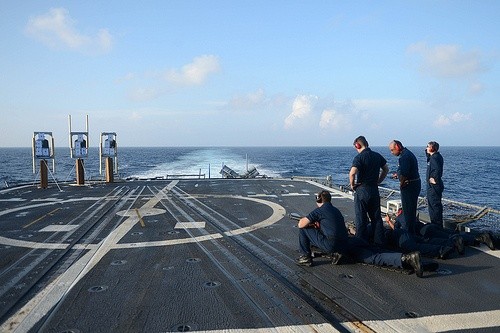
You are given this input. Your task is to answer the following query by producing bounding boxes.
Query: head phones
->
[431,143,436,152]
[316,190,324,202]
[398,209,403,215]
[394,140,401,151]
[354,138,361,149]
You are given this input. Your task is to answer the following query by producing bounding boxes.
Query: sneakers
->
[440,246,453,260]
[332,252,342,264]
[475,233,494,250]
[410,251,423,278]
[422,261,440,272]
[295,256,312,266]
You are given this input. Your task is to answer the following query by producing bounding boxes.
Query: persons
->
[349,136,389,242]
[384,208,495,260]
[294,190,349,266]
[424,141,445,232]
[345,234,424,278]
[389,139,421,218]
